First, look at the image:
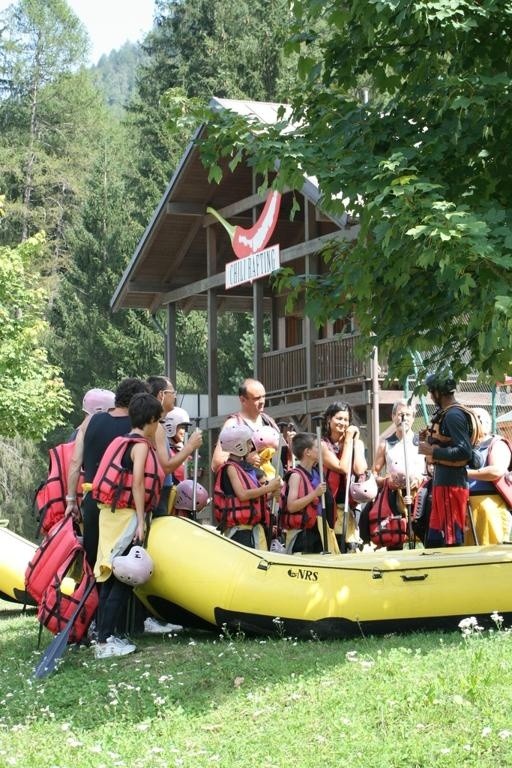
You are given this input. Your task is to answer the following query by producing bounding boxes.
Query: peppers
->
[206,188,282,259]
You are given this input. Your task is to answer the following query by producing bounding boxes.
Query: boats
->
[82,498,512,635]
[0,525,75,614]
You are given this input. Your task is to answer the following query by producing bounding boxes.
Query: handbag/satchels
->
[493,470,512,510]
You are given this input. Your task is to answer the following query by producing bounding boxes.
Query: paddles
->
[35,578,96,681]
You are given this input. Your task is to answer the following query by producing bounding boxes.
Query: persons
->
[210,375,511,556]
[64,375,208,660]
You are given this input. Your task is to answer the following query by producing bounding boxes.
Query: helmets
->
[252,425,281,452]
[81,388,116,414]
[161,407,193,438]
[467,406,492,438]
[427,370,458,393]
[349,470,378,504]
[173,479,208,513]
[110,545,153,587]
[220,424,253,458]
[271,539,285,553]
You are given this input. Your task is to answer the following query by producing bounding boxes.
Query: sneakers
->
[144,616,183,634]
[91,635,136,660]
